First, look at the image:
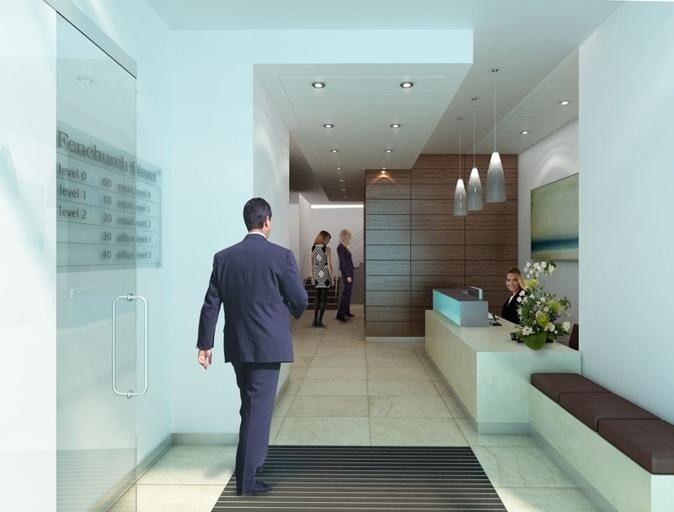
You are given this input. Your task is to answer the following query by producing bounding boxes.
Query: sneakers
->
[346,313,355,317]
[336,315,347,321]
[312,320,325,327]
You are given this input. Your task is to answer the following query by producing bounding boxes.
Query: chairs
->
[569,324,579,351]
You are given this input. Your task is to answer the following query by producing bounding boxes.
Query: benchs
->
[529,372,674,512]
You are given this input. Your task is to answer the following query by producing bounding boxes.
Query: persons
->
[501,266,531,327]
[336,228,355,322]
[310,230,335,328]
[194,196,309,497]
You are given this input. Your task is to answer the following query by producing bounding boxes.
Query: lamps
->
[485,68,506,203]
[467,97,483,211]
[453,117,467,217]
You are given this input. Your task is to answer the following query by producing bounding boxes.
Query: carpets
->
[211,445,508,512]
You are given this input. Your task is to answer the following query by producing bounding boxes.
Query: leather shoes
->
[237,480,272,496]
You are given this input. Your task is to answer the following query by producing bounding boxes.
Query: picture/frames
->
[530,172,579,261]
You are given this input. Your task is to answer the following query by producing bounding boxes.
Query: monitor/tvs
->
[468,285,484,299]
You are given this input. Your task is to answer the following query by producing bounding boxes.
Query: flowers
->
[518,261,572,350]
